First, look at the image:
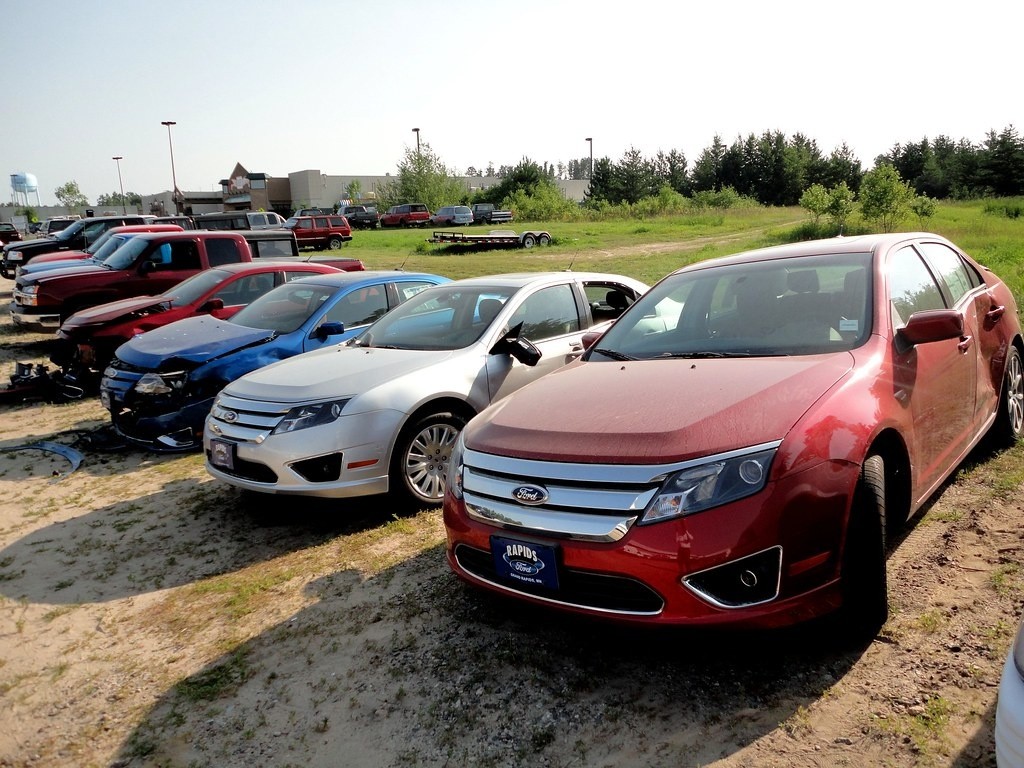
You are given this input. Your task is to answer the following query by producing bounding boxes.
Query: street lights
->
[586,136,593,200]
[162,119,183,216]
[112,156,128,214]
[411,127,422,154]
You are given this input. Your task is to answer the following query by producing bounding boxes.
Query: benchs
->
[589,291,630,326]
[776,270,843,325]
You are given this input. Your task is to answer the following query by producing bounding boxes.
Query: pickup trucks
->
[380,203,430,228]
[13,224,366,316]
[337,206,380,230]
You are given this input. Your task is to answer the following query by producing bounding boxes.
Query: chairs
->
[829,268,867,330]
[719,284,784,339]
[458,299,503,340]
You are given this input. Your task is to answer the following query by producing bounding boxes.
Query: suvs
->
[282,215,353,251]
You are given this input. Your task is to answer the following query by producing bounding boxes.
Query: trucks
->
[151,212,253,230]
[472,203,513,225]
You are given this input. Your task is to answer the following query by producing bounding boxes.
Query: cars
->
[203,270,709,513]
[0,222,24,268]
[55,263,350,391]
[430,206,474,226]
[35,219,76,238]
[29,221,43,234]
[451,228,1024,630]
[247,213,281,230]
[278,215,287,226]
[97,268,455,453]
[45,216,64,228]
[65,215,86,222]
[138,215,158,224]
[0,214,150,280]
[293,208,324,216]
